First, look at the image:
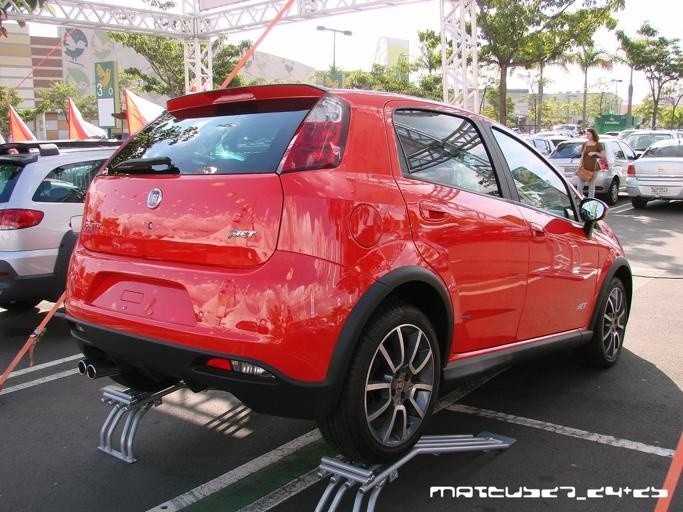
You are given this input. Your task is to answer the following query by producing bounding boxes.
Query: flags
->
[6,102,38,143]
[120,86,165,137]
[65,96,106,140]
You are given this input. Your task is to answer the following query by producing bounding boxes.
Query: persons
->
[574,129,606,200]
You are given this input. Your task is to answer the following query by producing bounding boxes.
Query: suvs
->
[0,137,241,314]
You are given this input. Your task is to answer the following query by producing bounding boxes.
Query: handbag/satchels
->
[575,168,593,182]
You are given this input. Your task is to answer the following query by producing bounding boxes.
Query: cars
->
[64,81,633,464]
[512,121,683,210]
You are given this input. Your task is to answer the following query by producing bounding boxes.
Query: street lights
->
[316,26,351,88]
